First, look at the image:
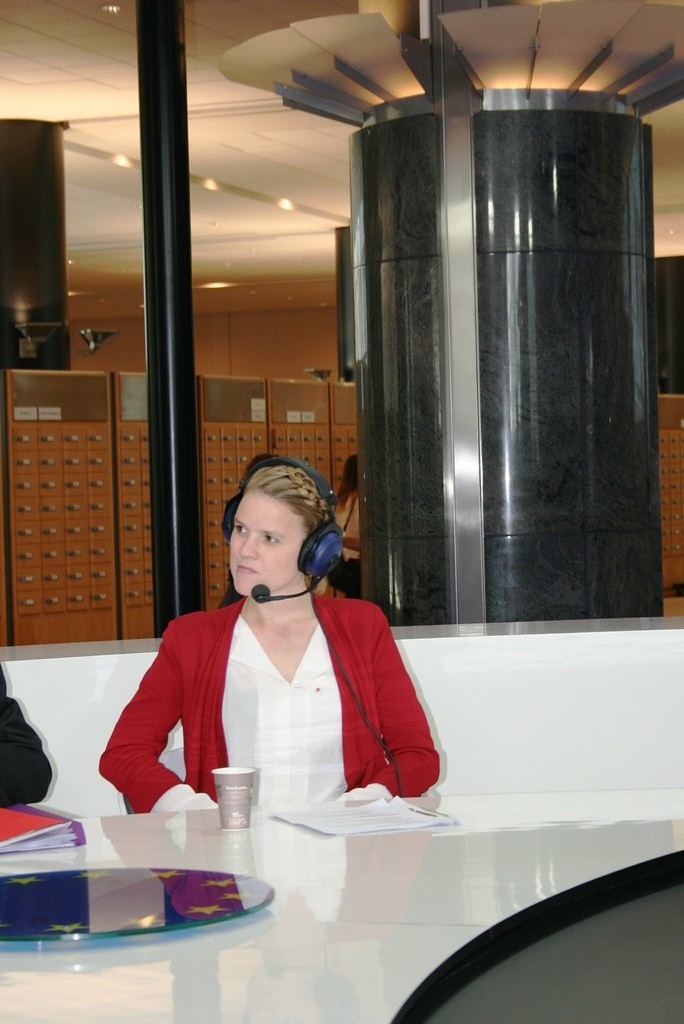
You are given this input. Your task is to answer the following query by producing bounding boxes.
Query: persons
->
[97,456,441,813]
[100,814,431,1024]
[0,664,54,809]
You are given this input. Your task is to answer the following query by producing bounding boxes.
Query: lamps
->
[75,328,120,360]
[17,321,61,358]
[306,369,332,383]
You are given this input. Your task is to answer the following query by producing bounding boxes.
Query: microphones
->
[252,575,325,603]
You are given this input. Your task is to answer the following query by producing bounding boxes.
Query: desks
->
[0,788,684,1024]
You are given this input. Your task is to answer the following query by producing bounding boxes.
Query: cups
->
[211,767,256,831]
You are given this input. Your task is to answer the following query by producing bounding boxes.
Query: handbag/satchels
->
[328,550,358,594]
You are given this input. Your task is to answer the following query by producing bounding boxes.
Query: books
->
[0,803,66,844]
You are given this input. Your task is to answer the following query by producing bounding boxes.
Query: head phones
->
[220,457,344,580]
